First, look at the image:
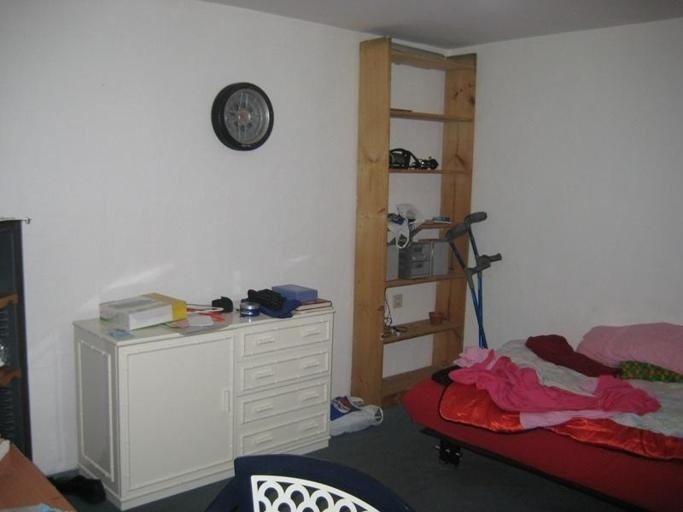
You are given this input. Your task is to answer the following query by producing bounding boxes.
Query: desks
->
[0,442,77,512]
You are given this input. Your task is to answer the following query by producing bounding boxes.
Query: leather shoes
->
[51,473,106,504]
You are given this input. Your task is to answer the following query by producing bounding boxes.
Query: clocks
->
[211,82,274,150]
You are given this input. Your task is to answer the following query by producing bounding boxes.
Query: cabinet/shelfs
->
[351,37,477,409]
[74,297,335,512]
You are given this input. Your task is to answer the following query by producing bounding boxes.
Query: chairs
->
[202,454,417,512]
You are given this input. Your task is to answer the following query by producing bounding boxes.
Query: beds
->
[401,358,683,512]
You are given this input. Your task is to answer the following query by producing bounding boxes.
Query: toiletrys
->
[239,301,260,316]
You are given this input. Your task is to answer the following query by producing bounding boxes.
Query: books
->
[293,297,332,312]
